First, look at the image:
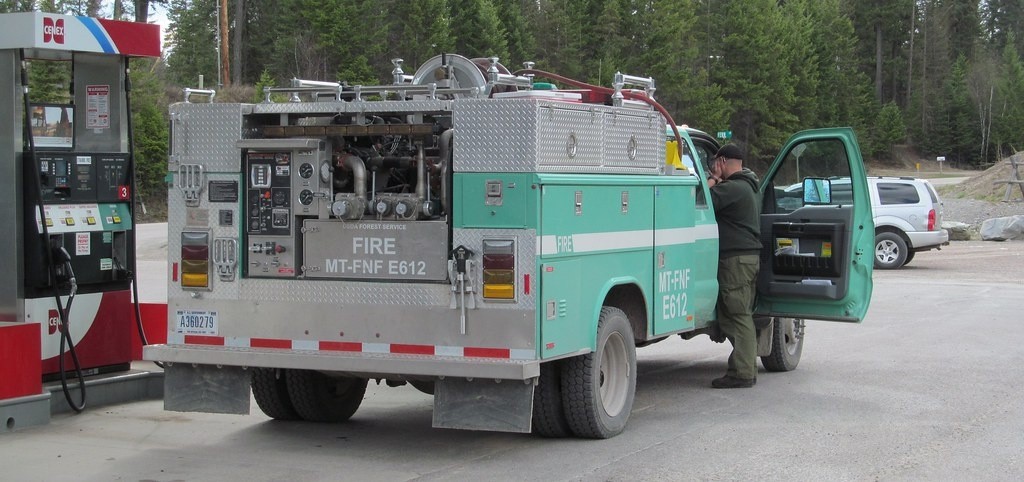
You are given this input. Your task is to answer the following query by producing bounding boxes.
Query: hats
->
[715,143,743,159]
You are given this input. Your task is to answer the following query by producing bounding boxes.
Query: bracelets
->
[708,176,719,185]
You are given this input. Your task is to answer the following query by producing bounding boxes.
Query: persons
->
[697,143,763,388]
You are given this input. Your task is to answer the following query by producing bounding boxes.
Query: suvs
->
[780,175,949,271]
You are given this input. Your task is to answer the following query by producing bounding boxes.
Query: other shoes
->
[712,375,757,388]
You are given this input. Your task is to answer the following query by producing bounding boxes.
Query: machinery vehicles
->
[141,45,876,441]
